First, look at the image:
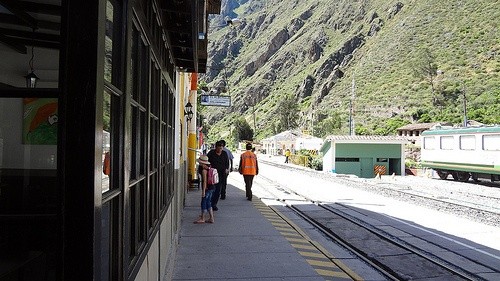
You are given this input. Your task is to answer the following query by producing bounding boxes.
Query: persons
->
[284,148,290,164]
[237,143,258,202]
[203,140,234,211]
[193,155,215,224]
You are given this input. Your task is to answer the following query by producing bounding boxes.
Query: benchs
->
[188,173,200,191]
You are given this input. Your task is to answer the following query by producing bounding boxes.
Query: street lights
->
[436,70,467,128]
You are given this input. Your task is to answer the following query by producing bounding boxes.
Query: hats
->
[196,156,210,164]
[246,144,252,149]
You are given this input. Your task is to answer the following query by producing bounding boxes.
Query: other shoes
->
[221,196,226,200]
[213,208,218,211]
[247,198,252,200]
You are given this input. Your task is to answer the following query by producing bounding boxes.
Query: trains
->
[419,124,500,187]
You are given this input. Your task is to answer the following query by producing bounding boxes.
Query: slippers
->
[193,220,205,224]
[205,219,214,223]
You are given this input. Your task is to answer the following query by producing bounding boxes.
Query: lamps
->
[184,101,194,123]
[25,25,41,88]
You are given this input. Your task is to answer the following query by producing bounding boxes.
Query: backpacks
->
[207,168,219,185]
